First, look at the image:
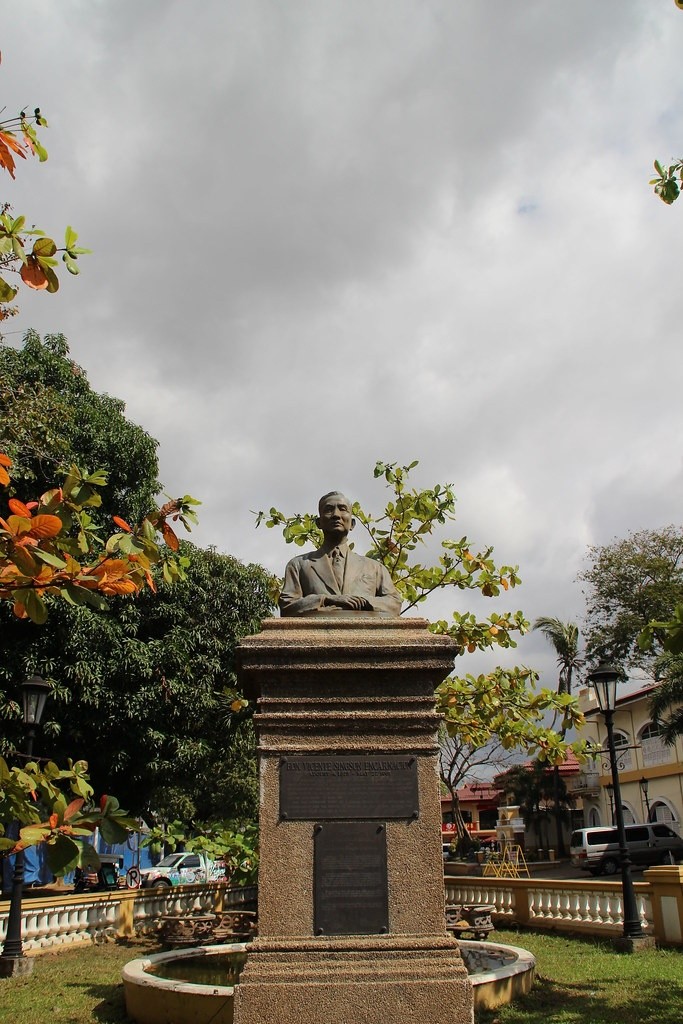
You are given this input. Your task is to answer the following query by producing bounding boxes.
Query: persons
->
[278,491,402,617]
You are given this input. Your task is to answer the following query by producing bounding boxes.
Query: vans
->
[570,821,683,875]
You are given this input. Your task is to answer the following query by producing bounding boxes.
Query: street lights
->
[0,664,56,979]
[586,659,648,939]
[606,782,616,827]
[639,776,652,823]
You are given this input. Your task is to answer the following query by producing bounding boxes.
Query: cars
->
[443,843,455,857]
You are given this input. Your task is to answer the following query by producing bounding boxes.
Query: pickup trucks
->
[139,852,226,888]
